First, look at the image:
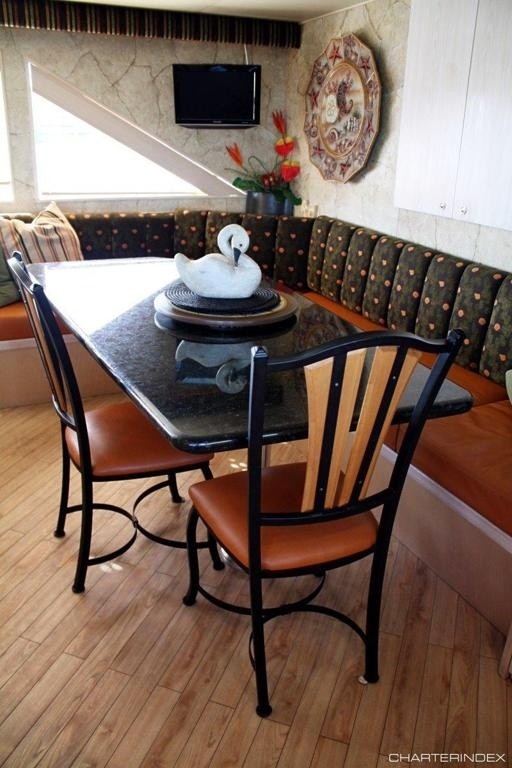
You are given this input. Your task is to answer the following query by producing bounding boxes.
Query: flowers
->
[224,108,303,207]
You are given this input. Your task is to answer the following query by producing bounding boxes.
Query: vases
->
[244,191,293,219]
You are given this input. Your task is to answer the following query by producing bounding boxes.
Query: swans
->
[173,223,263,299]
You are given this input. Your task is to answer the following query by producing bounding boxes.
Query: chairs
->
[10,264,227,597]
[179,326,465,719]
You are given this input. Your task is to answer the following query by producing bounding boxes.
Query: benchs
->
[1,207,510,636]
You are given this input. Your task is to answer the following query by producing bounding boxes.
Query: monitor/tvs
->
[172,63,261,129]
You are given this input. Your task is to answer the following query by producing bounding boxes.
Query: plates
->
[300,33,384,185]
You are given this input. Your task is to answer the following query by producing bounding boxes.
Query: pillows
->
[1,198,87,265]
[1,253,23,308]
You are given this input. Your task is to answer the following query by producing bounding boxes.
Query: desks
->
[16,252,476,582]
